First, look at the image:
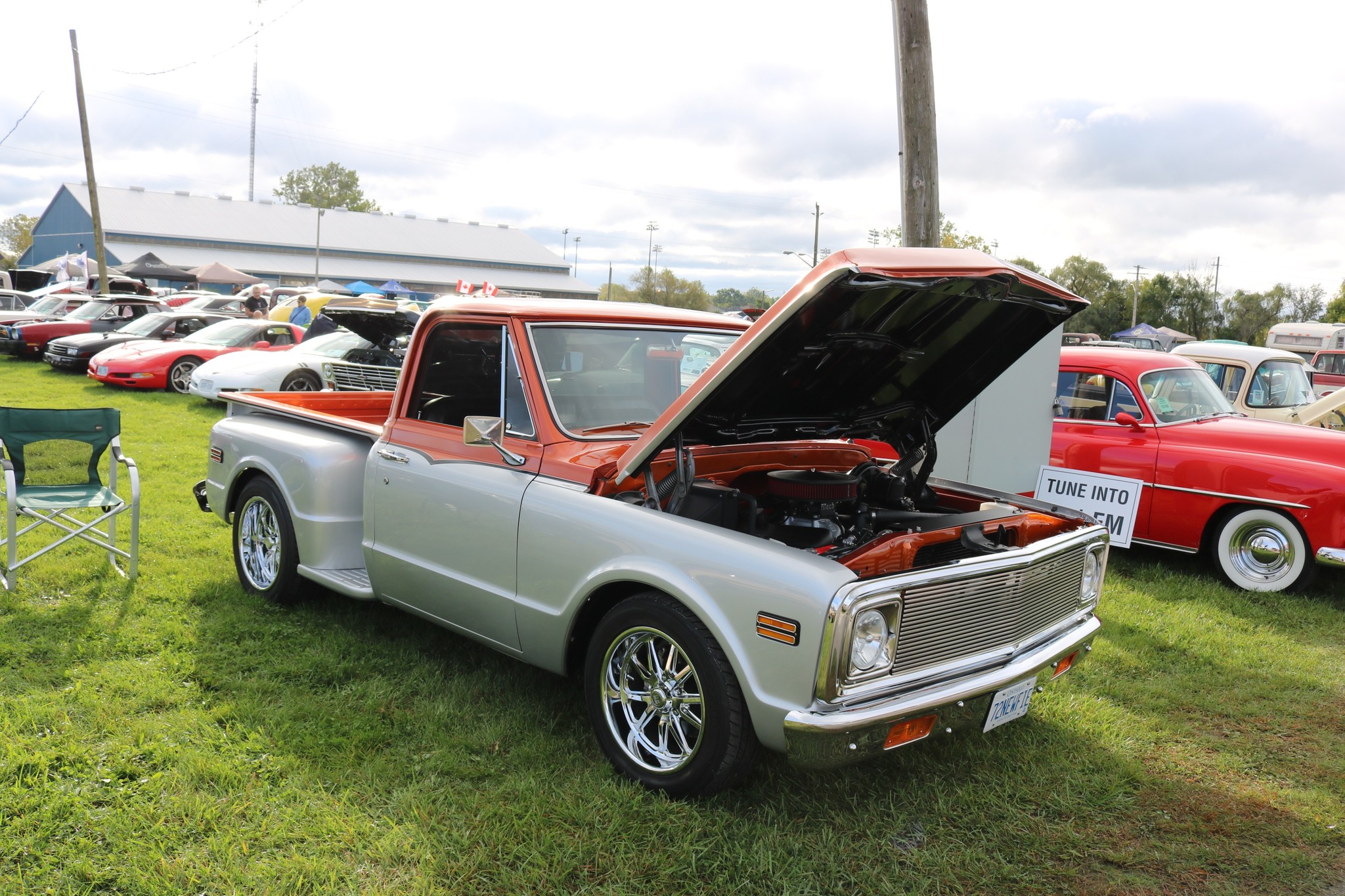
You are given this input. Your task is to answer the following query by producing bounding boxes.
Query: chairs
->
[1325,364,1337,372]
[175,323,189,338]
[1085,406,1125,422]
[0,407,140,590]
[274,333,290,345]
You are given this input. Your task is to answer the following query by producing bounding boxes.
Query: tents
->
[114,252,196,291]
[188,260,263,292]
[379,279,417,302]
[309,280,352,296]
[343,281,386,298]
[1109,323,1197,346]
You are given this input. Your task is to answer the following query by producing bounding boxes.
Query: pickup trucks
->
[1024,319,1345,595]
[193,247,1110,800]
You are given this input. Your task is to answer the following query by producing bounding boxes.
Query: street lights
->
[754,287,774,309]
[562,229,569,260]
[652,244,663,304]
[646,221,659,287]
[868,228,880,248]
[574,236,581,278]
[298,185,321,288]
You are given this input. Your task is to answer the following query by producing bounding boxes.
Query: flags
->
[483,281,498,296]
[68,251,88,279]
[55,252,70,282]
[456,280,474,294]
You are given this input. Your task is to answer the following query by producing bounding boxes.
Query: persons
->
[289,296,310,326]
[245,287,269,320]
[254,311,262,320]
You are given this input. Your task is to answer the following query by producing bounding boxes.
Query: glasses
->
[297,301,300,303]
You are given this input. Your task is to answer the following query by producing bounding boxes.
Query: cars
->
[0,269,767,400]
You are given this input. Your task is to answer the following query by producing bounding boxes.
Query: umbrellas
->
[26,253,121,282]
